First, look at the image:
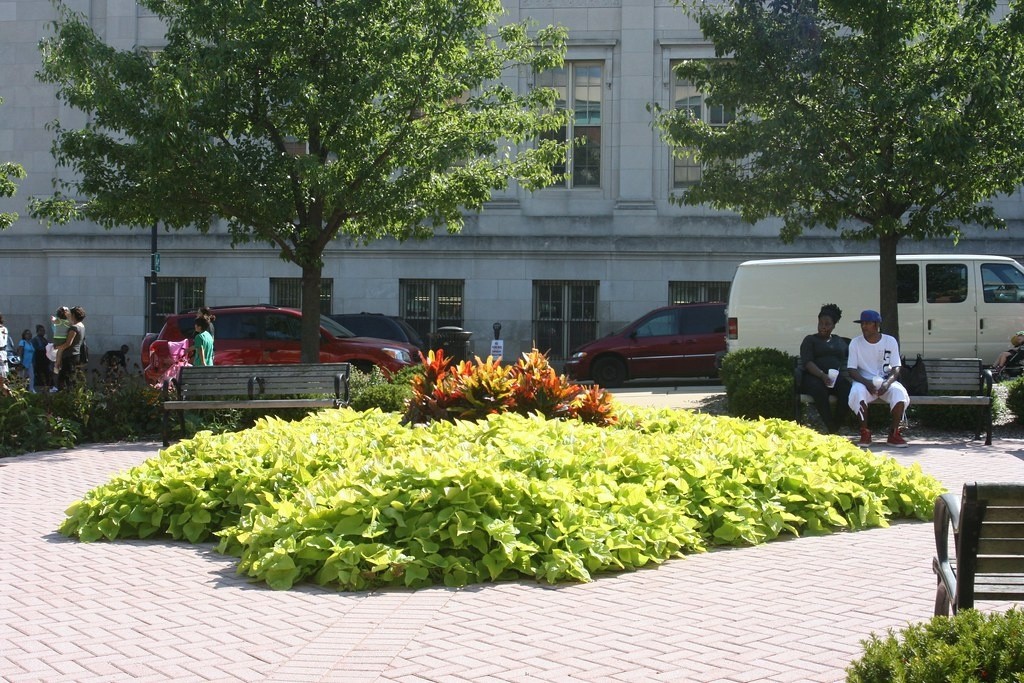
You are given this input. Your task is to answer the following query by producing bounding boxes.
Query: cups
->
[827,368,840,388]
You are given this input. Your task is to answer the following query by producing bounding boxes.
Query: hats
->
[853,310,882,323]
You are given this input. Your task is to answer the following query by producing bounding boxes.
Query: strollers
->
[992,345,1024,384]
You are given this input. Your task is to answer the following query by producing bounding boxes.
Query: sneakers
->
[857,429,872,447]
[886,428,908,447]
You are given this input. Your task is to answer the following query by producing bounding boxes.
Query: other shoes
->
[49,387,58,393]
[31,388,36,393]
[53,367,62,374]
[989,365,1002,372]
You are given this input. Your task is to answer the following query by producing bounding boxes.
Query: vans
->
[728,254,1024,370]
[326,311,426,361]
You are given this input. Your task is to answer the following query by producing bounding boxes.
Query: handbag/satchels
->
[79,337,89,363]
[8,340,25,369]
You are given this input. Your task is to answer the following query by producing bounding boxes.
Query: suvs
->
[562,299,729,388]
[138,303,421,390]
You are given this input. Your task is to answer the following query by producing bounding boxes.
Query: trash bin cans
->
[427,326,472,365]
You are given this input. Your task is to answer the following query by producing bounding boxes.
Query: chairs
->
[932,481,1024,619]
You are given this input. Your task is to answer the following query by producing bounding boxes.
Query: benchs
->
[158,363,350,446]
[794,355,993,445]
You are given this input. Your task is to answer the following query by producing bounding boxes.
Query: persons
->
[132,363,143,374]
[801,305,852,434]
[184,306,216,366]
[0,311,9,389]
[18,330,37,393]
[100,344,129,375]
[989,330,1024,371]
[32,325,54,386]
[49,306,87,391]
[847,310,910,447]
